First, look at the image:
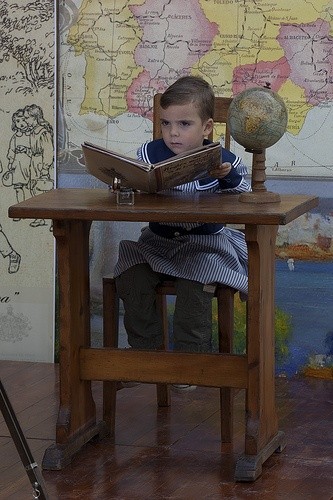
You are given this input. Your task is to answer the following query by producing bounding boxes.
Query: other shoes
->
[169,384,198,393]
[121,381,141,389]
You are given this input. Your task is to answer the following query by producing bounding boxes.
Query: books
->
[80,139,224,200]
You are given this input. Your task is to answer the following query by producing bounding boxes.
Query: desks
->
[9,185,322,481]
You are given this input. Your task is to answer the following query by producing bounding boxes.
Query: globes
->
[227,87,289,202]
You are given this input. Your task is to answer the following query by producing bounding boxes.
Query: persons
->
[106,75,253,395]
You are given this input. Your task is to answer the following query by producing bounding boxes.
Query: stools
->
[94,258,245,451]
